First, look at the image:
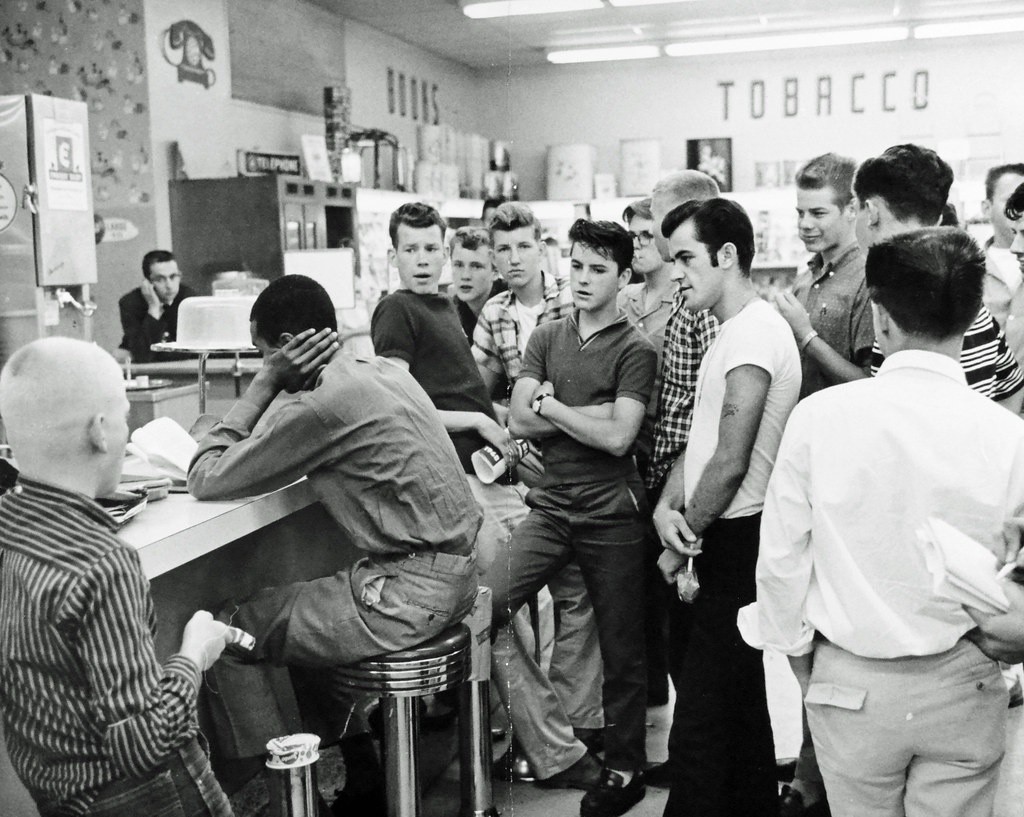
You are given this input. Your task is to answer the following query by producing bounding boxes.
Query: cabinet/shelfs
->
[171,176,363,295]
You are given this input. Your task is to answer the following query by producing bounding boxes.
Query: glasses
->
[629,232,653,246]
[150,272,182,284]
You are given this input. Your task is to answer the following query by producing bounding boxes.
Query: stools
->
[318,624,472,817]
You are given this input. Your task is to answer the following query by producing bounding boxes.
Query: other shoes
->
[327,768,385,817]
[255,786,324,817]
[644,760,672,789]
[1008,675,1023,708]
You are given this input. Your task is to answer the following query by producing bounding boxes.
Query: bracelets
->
[799,330,818,350]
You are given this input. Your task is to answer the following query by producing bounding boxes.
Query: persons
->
[186,273,485,817]
[373,204,605,790]
[448,142,1024,817]
[754,229,1024,817]
[651,193,803,817]
[0,337,235,817]
[118,250,195,365]
[486,216,659,817]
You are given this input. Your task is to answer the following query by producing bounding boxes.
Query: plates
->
[126,380,174,390]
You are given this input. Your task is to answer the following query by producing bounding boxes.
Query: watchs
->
[532,393,551,414]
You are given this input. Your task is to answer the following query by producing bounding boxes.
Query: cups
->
[413,124,519,200]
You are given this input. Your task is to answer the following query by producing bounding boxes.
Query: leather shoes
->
[580,770,646,817]
[570,727,605,751]
[536,750,605,789]
[779,784,831,817]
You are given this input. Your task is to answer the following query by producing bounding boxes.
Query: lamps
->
[344,128,398,190]
[458,0,1024,65]
